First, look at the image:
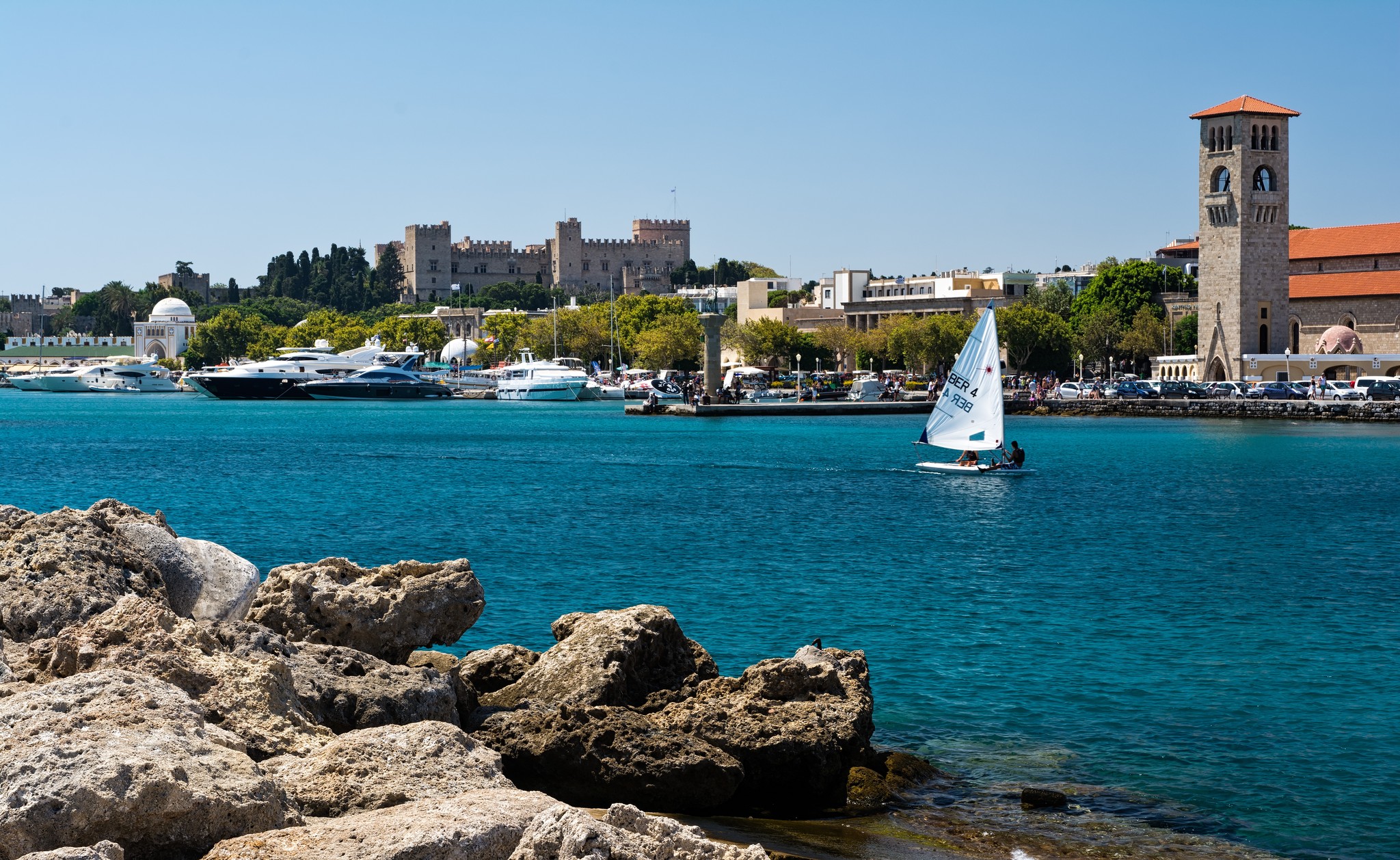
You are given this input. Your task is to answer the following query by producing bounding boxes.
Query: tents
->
[618,368,705,387]
[723,366,766,389]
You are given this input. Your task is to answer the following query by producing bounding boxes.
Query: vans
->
[1354,376,1400,401]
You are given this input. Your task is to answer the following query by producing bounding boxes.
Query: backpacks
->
[689,383,693,391]
[932,385,935,390]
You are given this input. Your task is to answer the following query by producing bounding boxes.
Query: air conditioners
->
[1085,267,1089,271]
[795,304,800,308]
[1080,266,1084,271]
[957,269,961,273]
[962,269,967,273]
[788,303,793,308]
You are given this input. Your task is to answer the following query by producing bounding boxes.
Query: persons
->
[978,441,1026,474]
[1310,373,1327,401]
[396,361,399,364]
[1002,360,1147,406]
[956,450,978,467]
[501,376,507,381]
[322,370,337,381]
[810,370,947,404]
[588,375,769,414]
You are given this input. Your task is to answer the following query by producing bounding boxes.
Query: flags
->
[671,189,675,193]
[484,336,500,344]
[403,333,407,342]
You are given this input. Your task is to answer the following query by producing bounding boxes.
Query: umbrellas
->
[595,373,613,389]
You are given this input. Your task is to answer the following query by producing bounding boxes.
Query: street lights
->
[1194,345,1197,355]
[819,359,821,377]
[764,358,767,366]
[608,359,612,371]
[1079,354,1083,379]
[1285,347,1291,382]
[816,357,819,380]
[1109,356,1113,385]
[870,358,873,379]
[598,360,601,367]
[796,354,801,403]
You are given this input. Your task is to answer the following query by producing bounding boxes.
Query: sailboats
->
[911,296,1037,476]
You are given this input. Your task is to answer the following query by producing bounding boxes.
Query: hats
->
[1031,391,1035,394]
[695,392,699,395]
[690,380,693,382]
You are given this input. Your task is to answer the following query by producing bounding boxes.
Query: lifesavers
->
[624,374,627,380]
[647,374,652,380]
[491,375,497,381]
[453,365,458,370]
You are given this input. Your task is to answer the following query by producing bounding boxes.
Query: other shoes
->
[926,400,929,402]
[892,400,896,402]
[812,401,814,403]
[814,401,817,403]
[977,466,985,473]
[991,458,995,466]
[899,400,902,401]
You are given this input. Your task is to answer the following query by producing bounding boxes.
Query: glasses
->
[1322,375,1324,376]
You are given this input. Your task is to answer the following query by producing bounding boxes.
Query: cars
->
[1087,383,1117,399]
[1366,381,1400,401]
[1019,376,1029,381]
[1003,375,1017,383]
[906,373,922,381]
[1056,382,1093,400]
[1307,380,1360,401]
[1261,381,1309,400]
[1340,380,1356,388]
[1107,380,1316,400]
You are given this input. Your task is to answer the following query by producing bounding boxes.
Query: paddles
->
[946,458,986,463]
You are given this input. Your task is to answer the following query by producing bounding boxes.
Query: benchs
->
[1018,392,1031,398]
[699,396,729,404]
[878,393,898,402]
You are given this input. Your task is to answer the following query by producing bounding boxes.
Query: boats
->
[0,273,909,416]
